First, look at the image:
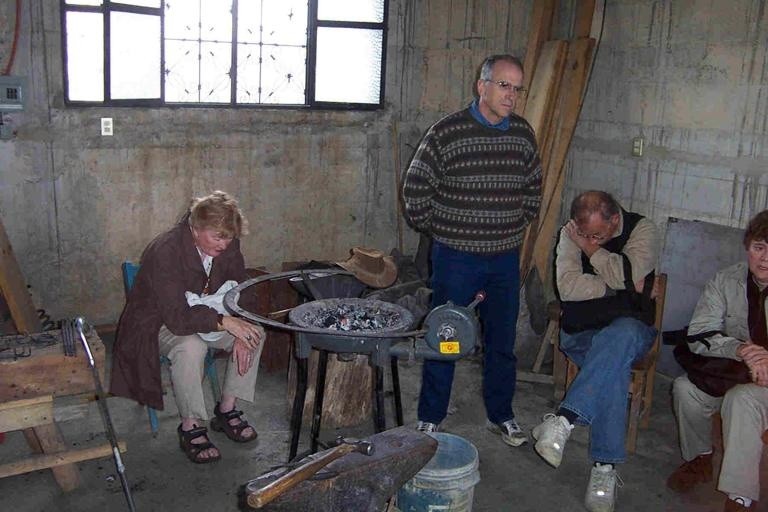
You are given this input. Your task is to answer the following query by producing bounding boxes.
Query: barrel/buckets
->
[397,432,481,512]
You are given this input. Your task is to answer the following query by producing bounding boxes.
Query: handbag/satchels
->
[677,326,753,384]
[561,267,657,326]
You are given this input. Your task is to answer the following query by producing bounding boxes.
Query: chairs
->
[566,273,667,456]
[122,262,222,431]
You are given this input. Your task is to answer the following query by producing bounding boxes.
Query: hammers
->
[245,434,374,507]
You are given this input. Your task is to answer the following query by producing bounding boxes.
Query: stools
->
[532,300,560,373]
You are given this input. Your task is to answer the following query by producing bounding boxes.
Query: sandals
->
[211,401,258,444]
[177,420,222,463]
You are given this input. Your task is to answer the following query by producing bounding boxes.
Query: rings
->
[248,335,253,340]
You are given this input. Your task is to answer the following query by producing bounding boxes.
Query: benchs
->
[0,325,128,494]
[669,396,768,446]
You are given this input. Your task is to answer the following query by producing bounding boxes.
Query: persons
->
[109,187,268,463]
[664,208,767,510]
[530,188,663,510]
[402,53,548,450]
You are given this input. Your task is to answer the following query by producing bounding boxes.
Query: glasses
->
[484,77,527,99]
[575,224,612,241]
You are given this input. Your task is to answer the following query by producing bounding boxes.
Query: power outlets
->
[632,137,643,157]
[101,117,113,136]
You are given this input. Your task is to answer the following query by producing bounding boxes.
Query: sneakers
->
[584,465,617,512]
[668,446,715,492]
[532,415,569,470]
[486,417,527,447]
[724,496,755,511]
[417,417,442,436]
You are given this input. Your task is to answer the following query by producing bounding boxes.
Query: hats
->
[336,247,397,288]
